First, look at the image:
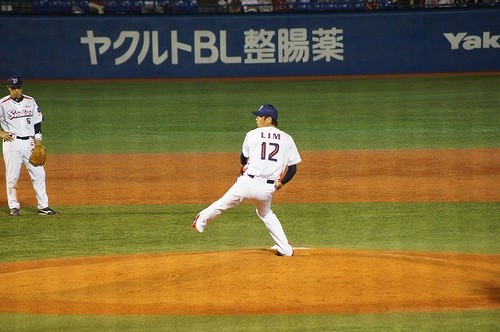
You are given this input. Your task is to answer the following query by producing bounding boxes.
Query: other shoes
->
[9,208,20,216]
[37,208,59,216]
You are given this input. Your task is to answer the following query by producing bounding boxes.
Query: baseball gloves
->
[29,145,47,167]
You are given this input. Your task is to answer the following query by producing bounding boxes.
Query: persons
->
[193,103,302,256]
[88,0,500,13]
[0,76,60,215]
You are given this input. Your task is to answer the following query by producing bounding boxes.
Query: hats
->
[252,103,278,120]
[7,75,24,88]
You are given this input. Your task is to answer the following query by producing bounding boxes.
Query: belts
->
[248,175,275,184]
[9,135,30,140]
